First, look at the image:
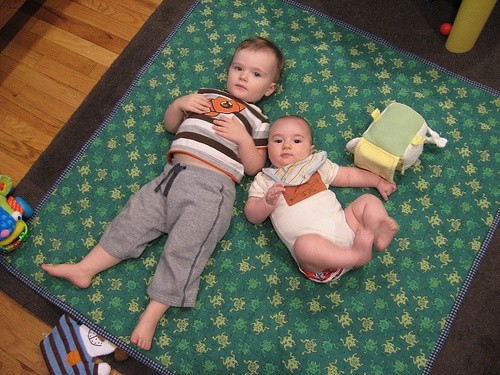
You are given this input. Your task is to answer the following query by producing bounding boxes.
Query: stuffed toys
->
[40,313,129,375]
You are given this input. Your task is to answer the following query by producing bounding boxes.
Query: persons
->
[40,36,285,350]
[244,115,401,283]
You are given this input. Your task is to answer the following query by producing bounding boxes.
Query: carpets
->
[0,1,500,375]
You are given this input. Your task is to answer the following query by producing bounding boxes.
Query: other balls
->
[440,23,451,36]
